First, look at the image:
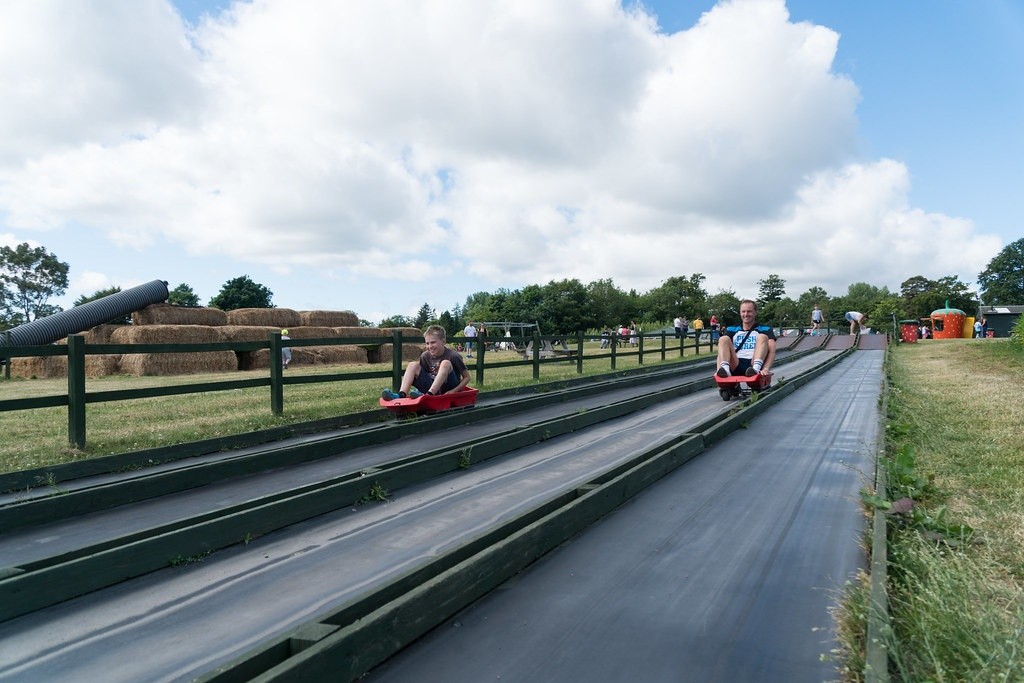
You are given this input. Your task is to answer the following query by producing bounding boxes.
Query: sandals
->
[409,390,423,398]
[382,389,399,401]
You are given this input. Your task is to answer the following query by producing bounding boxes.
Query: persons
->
[973,315,988,338]
[717,300,777,376]
[281,329,291,368]
[844,311,868,335]
[495,331,517,351]
[673,314,726,339]
[478,322,487,357]
[464,320,476,358]
[918,324,930,339]
[600,320,639,349]
[456,342,463,352]
[811,304,824,336]
[779,314,788,337]
[382,326,470,401]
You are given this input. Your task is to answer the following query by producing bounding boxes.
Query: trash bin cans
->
[986,328,995,337]
[899,320,920,343]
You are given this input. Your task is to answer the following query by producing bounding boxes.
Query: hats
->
[281,329,288,335]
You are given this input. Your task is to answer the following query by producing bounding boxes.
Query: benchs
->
[516,349,578,366]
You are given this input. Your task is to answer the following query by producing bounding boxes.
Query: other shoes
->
[810,332,815,336]
[466,354,473,358]
[283,363,288,369]
[717,367,728,378]
[818,333,822,336]
[746,367,757,376]
